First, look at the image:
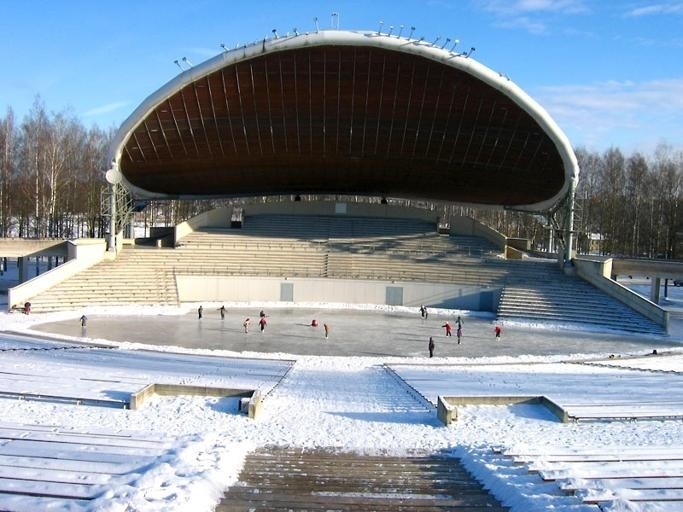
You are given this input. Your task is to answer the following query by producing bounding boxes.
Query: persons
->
[457,328,462,345]
[22,302,31,314]
[258,319,266,332]
[217,306,227,320]
[245,319,250,332]
[80,315,88,327]
[442,322,452,337]
[420,305,425,317]
[456,316,464,327]
[260,310,266,318]
[240,320,247,332]
[198,306,202,319]
[424,308,428,319]
[493,325,502,342]
[428,337,435,357]
[324,324,328,338]
